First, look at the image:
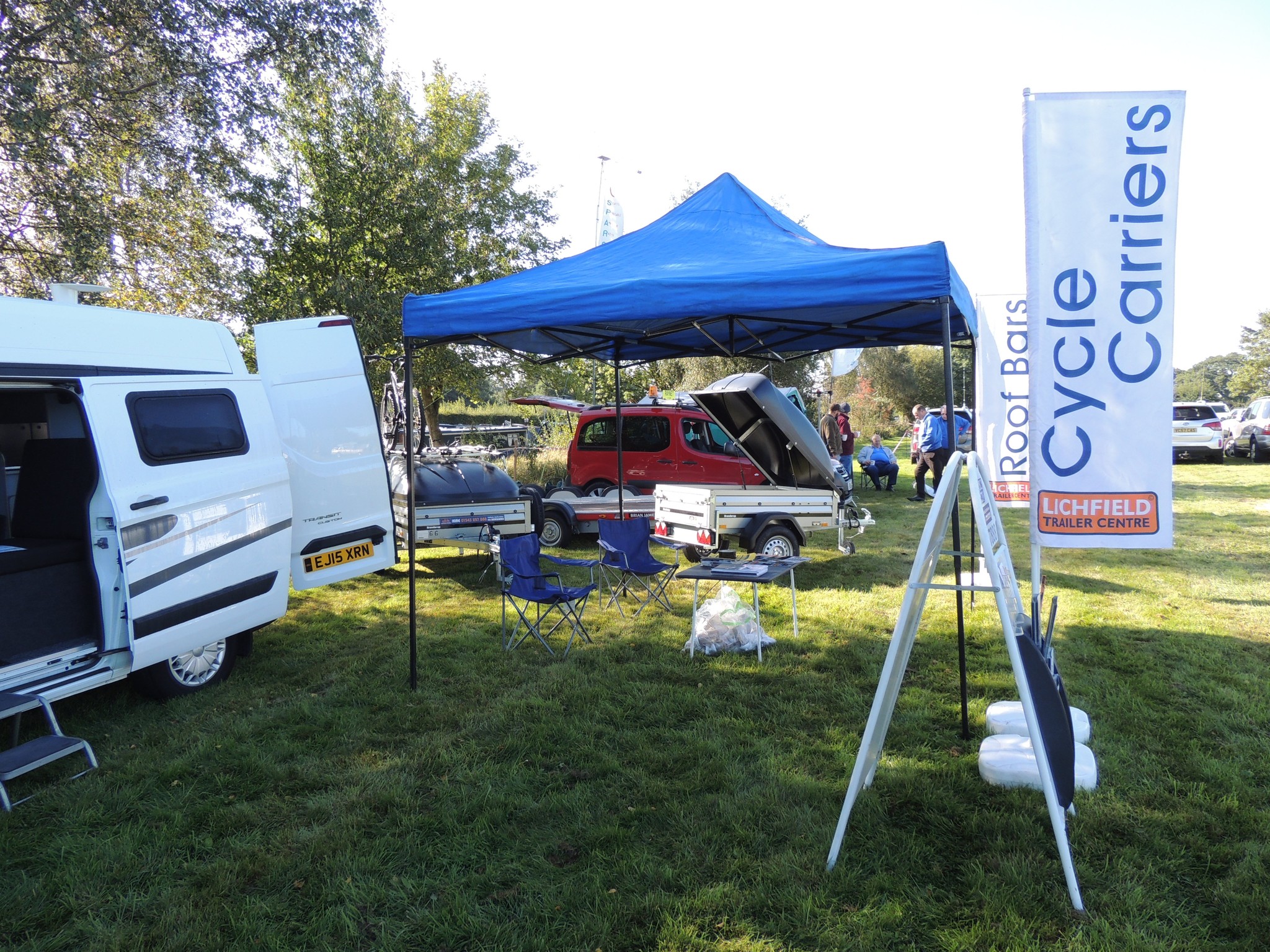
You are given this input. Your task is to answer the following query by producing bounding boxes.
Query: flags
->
[976,294,1029,508]
[1020,90,1188,549]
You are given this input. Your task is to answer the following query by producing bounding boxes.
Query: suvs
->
[1195,400,1230,420]
[1172,401,1223,464]
[1224,395,1270,462]
[910,405,973,464]
[1220,407,1247,437]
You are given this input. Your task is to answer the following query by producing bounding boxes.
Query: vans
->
[0,281,400,719]
[637,385,853,504]
[509,395,771,498]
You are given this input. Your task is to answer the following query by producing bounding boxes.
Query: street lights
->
[962,362,967,409]
[1200,365,1207,400]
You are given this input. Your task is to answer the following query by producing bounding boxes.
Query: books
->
[710,563,768,577]
[700,555,749,567]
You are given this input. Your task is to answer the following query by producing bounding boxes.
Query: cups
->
[870,460,875,465]
[841,435,847,441]
[719,549,735,558]
[853,431,860,438]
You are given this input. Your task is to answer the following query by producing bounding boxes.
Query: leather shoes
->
[886,487,895,492]
[907,495,925,501]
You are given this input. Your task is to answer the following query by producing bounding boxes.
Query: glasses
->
[941,413,947,416]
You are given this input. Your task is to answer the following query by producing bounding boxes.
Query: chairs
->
[596,516,688,619]
[691,422,707,452]
[498,533,599,659]
[860,464,893,491]
[683,422,693,448]
[0,438,94,659]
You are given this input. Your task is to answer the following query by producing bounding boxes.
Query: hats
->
[828,403,846,412]
[840,402,850,413]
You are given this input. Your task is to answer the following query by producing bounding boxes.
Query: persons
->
[836,402,858,473]
[906,404,942,502]
[857,435,900,492]
[936,405,970,470]
[820,404,845,460]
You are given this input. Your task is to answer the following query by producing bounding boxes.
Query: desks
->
[676,560,804,664]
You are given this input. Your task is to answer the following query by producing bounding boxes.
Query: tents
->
[403,171,978,743]
